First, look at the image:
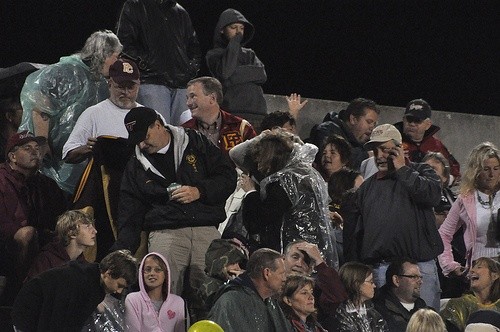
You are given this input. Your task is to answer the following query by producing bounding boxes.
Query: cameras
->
[167,182,183,201]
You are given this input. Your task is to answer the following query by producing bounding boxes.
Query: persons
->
[0,0,266,175]
[438,141,500,294]
[0,92,467,332]
[440,256,500,332]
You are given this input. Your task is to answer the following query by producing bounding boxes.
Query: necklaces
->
[202,112,221,130]
[476,189,496,209]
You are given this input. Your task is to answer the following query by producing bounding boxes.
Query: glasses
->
[406,117,430,124]
[396,274,424,283]
[365,277,378,286]
[142,268,164,274]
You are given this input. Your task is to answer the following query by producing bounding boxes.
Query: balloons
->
[188,320,224,332]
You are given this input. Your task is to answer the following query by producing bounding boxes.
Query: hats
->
[124,107,157,144]
[109,60,141,85]
[405,98,432,118]
[6,131,47,161]
[363,123,403,151]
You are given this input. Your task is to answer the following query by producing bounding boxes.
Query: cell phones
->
[389,144,401,162]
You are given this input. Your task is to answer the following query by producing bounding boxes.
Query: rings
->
[293,99,296,101]
[89,145,92,148]
[395,153,399,156]
